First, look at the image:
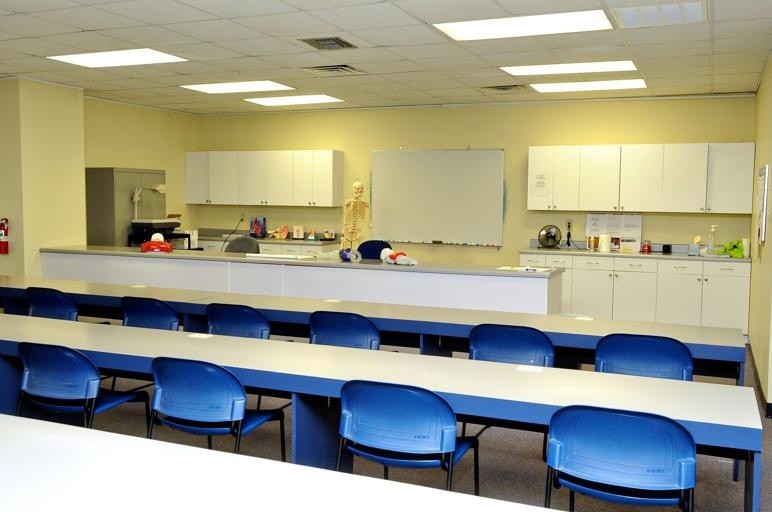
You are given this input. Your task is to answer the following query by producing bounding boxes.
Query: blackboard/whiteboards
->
[370,148,505,246]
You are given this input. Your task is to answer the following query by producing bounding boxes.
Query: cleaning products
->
[590,236,594,251]
[249,216,265,238]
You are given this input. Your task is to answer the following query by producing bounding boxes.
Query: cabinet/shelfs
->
[663,141,757,214]
[656,255,750,335]
[292,149,343,207]
[237,150,291,207]
[527,145,578,214]
[281,240,342,255]
[85,164,169,248]
[186,151,238,207]
[258,237,282,255]
[519,249,572,314]
[571,254,659,323]
[578,144,664,212]
[198,237,227,252]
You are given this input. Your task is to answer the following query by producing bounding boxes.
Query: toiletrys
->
[706,224,718,254]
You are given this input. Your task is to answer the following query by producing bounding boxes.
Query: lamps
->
[130,180,172,218]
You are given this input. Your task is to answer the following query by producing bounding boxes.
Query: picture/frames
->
[751,164,770,245]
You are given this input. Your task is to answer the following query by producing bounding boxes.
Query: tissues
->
[688,236,701,256]
[141,233,172,252]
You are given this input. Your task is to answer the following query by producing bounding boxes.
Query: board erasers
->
[432,240,442,244]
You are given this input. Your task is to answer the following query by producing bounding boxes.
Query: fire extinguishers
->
[0,218,9,256]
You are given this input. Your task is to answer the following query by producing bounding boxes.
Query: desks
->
[1,274,747,385]
[2,312,764,512]
[126,232,191,250]
[0,415,568,512]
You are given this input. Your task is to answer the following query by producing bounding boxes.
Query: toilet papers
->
[599,234,610,253]
[742,238,750,257]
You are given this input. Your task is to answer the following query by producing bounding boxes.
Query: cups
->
[599,234,612,252]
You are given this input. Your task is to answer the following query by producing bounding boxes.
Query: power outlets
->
[239,215,245,223]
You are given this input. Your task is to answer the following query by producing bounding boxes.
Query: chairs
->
[16,341,149,435]
[308,310,400,352]
[354,240,395,263]
[111,295,180,392]
[542,402,697,512]
[335,379,481,497]
[23,285,111,325]
[146,357,286,463]
[593,332,695,382]
[205,302,294,411]
[222,235,262,254]
[461,322,556,462]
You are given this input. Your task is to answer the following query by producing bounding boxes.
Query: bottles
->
[642,240,651,254]
[589,235,595,252]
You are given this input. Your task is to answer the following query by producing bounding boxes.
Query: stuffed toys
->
[380,248,417,266]
[339,247,363,263]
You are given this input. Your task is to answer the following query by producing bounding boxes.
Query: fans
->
[535,223,564,251]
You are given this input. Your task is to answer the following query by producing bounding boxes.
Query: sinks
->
[129,218,181,224]
